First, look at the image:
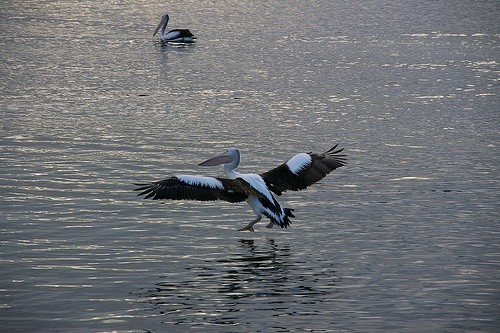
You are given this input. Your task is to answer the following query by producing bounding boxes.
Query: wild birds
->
[131,143,349,234]
[152,13,198,43]
